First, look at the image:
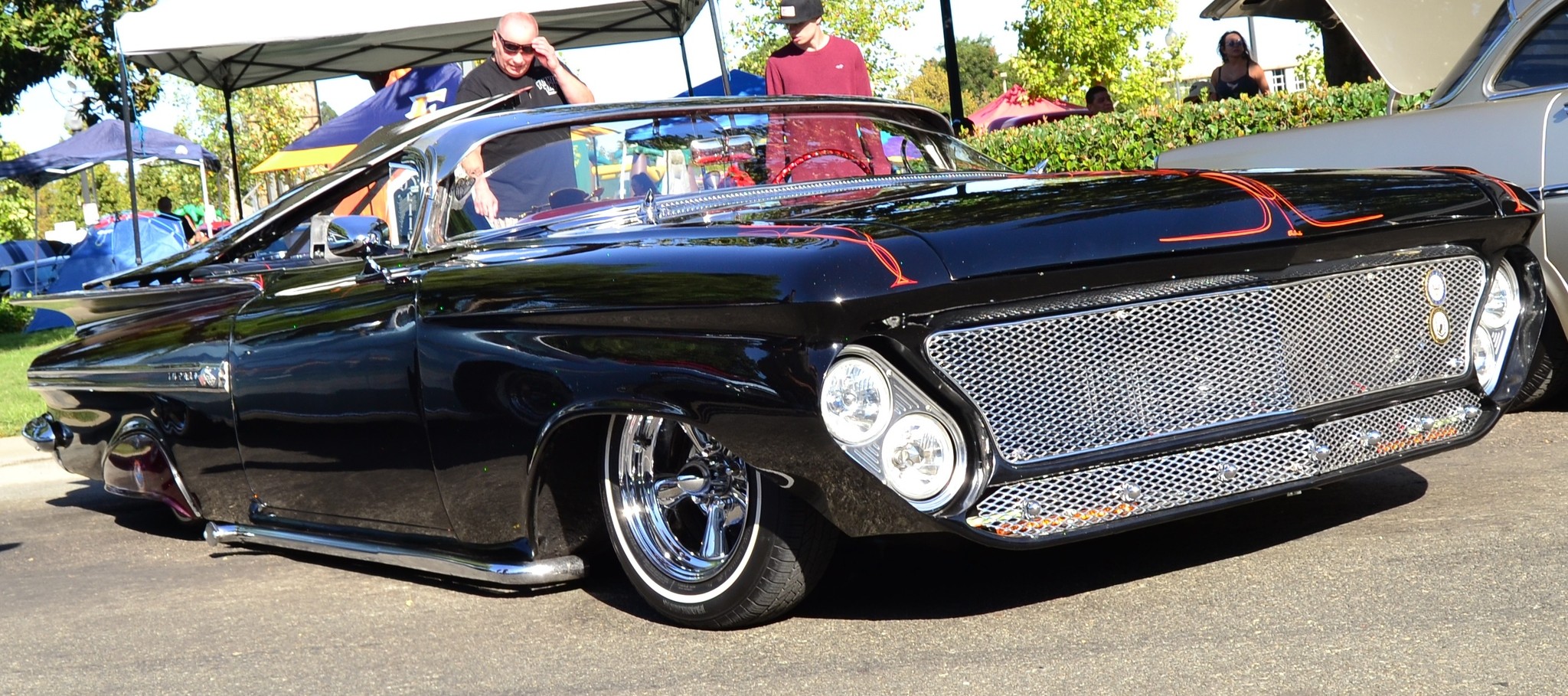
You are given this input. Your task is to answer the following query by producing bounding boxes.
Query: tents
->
[107,0,743,274]
[965,82,1085,133]
[624,67,772,195]
[247,62,463,174]
[0,115,224,294]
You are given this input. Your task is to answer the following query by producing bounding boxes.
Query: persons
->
[1209,31,1270,99]
[1182,81,1217,103]
[765,0,892,201]
[155,196,196,249]
[452,13,600,232]
[1085,86,1114,115]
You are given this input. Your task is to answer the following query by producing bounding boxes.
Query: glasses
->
[496,31,536,55]
[1224,38,1243,47]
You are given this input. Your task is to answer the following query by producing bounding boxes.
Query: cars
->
[1153,0,1568,412]
[26,94,1549,635]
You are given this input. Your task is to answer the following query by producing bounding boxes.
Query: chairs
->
[516,194,671,224]
[1494,69,1568,91]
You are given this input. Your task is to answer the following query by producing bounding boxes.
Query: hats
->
[768,1,825,25]
[1182,81,1217,103]
